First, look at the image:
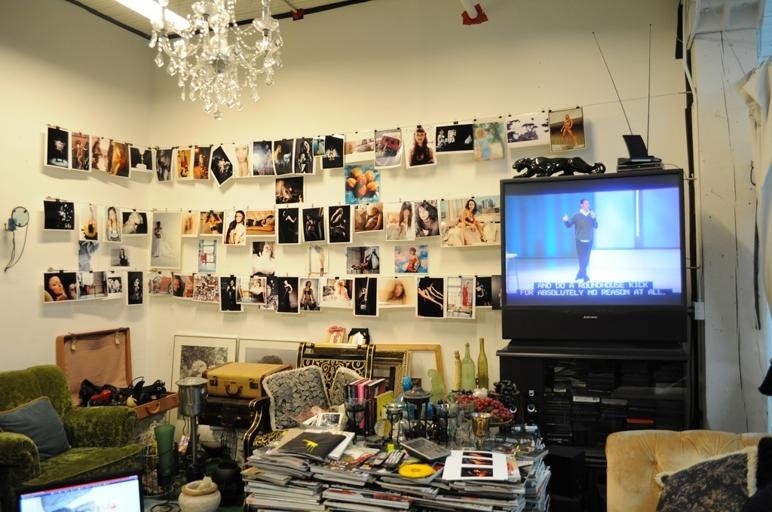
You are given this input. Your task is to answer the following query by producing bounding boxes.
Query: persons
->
[563,198,598,284]
[44,200,502,312]
[42,113,599,202]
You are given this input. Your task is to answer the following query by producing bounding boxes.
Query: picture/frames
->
[168,334,237,449]
[374,339,444,398]
[372,350,413,400]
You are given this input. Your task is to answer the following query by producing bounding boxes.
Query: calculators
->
[401,437,451,460]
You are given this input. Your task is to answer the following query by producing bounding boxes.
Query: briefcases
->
[58,327,180,421]
[198,362,286,429]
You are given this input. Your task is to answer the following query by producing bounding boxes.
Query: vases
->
[154,423,176,489]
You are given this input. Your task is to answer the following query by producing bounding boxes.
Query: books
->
[240,410,552,511]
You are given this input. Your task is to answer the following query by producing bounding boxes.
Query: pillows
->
[328,367,368,404]
[0,395,73,458]
[263,362,329,436]
[653,445,757,512]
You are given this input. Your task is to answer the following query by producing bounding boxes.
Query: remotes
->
[385,450,405,468]
[374,452,389,465]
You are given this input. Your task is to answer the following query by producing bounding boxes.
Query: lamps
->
[144,1,284,123]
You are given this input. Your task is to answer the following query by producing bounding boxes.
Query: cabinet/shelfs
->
[494,346,692,512]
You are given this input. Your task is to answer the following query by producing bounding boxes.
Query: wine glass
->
[471,410,493,449]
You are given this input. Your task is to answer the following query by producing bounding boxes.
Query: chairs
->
[604,427,771,512]
[243,341,381,463]
[0,364,148,512]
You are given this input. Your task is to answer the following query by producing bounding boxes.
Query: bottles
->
[462,341,475,389]
[478,337,488,389]
[396,376,432,420]
[453,350,462,392]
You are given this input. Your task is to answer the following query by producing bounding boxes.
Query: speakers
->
[523,359,546,426]
[505,374,524,424]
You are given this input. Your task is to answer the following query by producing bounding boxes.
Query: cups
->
[154,425,176,476]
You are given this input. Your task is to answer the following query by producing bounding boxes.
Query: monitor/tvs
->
[17,471,145,512]
[500,168,687,351]
